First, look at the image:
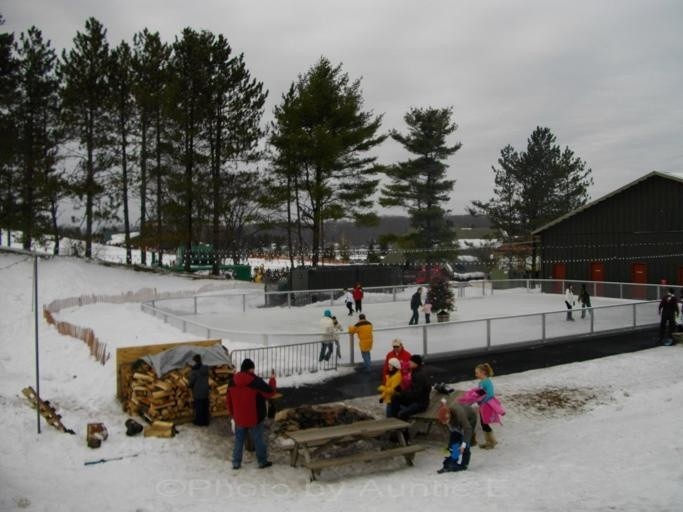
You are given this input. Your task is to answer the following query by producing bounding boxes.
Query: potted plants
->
[424,276,457,322]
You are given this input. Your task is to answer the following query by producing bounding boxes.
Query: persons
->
[408,287,423,325]
[376,339,497,474]
[564,284,576,321]
[318,310,336,361]
[352,282,364,313]
[658,288,679,344]
[187,354,210,426]
[578,284,591,318]
[331,316,343,359]
[348,314,372,368]
[342,287,354,315]
[422,299,433,323]
[225,358,277,469]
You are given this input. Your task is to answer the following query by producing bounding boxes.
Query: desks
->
[411,390,466,446]
[286,416,413,481]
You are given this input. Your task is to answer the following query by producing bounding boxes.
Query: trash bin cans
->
[236,265,250,281]
[490,269,509,289]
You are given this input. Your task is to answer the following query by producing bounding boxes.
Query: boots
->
[480,430,498,448]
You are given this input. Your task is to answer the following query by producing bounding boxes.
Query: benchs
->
[278,436,426,471]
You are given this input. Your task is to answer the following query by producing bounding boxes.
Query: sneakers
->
[258,460,273,469]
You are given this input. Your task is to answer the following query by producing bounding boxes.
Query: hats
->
[392,338,400,347]
[240,359,254,370]
[323,310,331,317]
[388,357,402,370]
